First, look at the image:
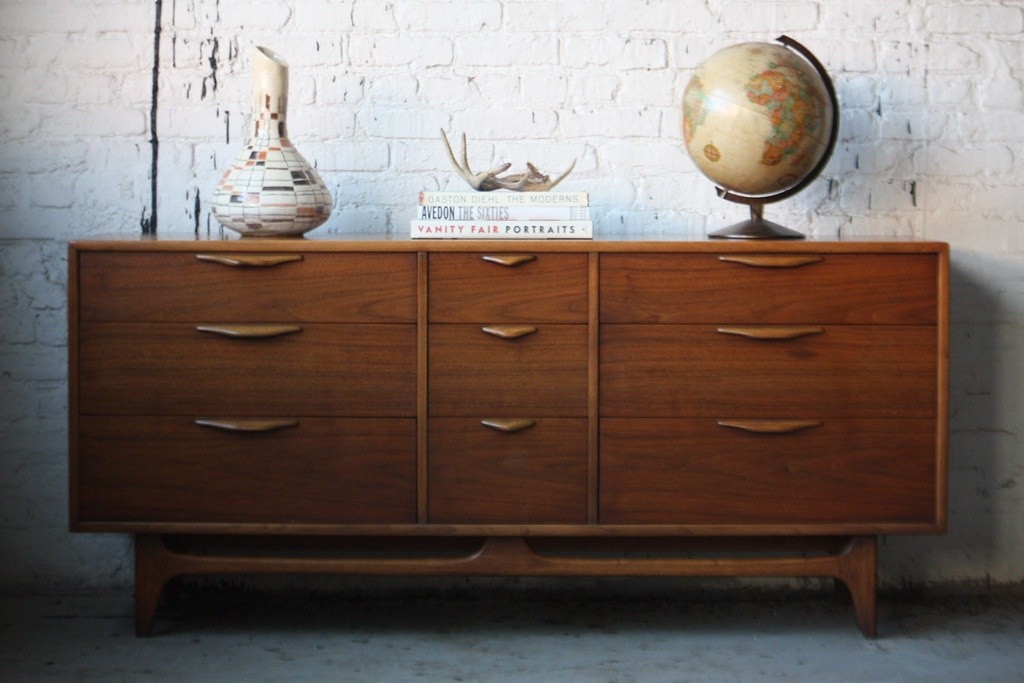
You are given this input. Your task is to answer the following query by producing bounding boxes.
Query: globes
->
[679,33,841,242]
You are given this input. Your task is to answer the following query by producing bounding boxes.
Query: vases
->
[210,45,333,238]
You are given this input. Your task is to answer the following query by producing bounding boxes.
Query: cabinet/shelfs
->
[598,251,937,527]
[66,247,418,538]
[421,251,591,537]
